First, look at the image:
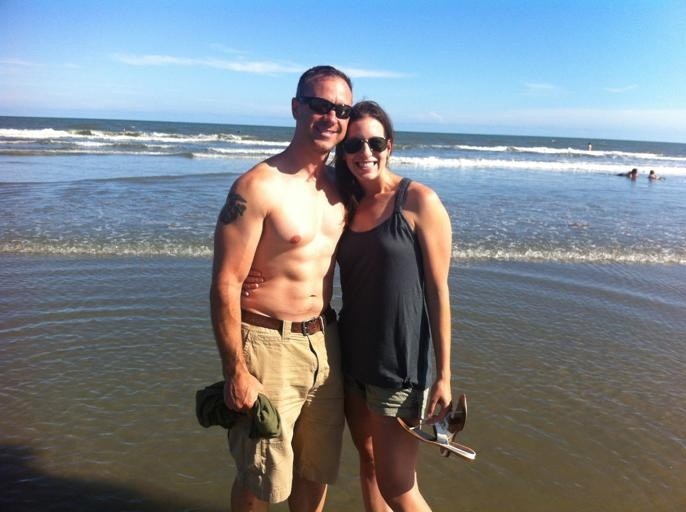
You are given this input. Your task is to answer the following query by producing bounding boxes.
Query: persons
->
[649,170,661,180]
[629,168,638,181]
[588,141,593,151]
[242,101,453,512]
[211,65,355,512]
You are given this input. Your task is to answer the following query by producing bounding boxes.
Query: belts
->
[241,309,337,336]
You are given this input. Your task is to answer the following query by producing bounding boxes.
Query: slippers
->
[396,393,477,461]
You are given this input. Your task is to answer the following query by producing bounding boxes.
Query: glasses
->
[298,96,352,119]
[343,136,388,154]
